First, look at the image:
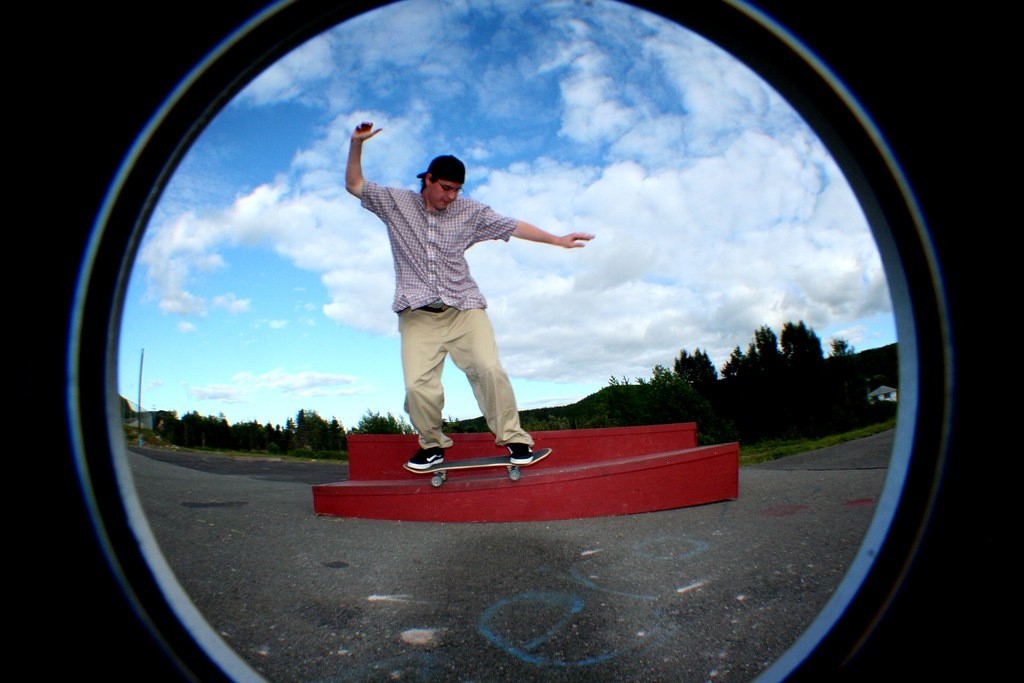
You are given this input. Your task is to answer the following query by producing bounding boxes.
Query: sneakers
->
[407,447,444,470]
[507,443,534,465]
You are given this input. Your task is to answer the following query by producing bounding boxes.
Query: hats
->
[417,155,465,183]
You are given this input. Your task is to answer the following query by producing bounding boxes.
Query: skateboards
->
[403,445,550,488]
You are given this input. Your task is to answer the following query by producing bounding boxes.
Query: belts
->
[418,305,444,312]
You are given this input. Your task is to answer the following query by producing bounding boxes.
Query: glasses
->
[436,180,464,196]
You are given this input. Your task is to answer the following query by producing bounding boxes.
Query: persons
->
[345,121,596,470]
